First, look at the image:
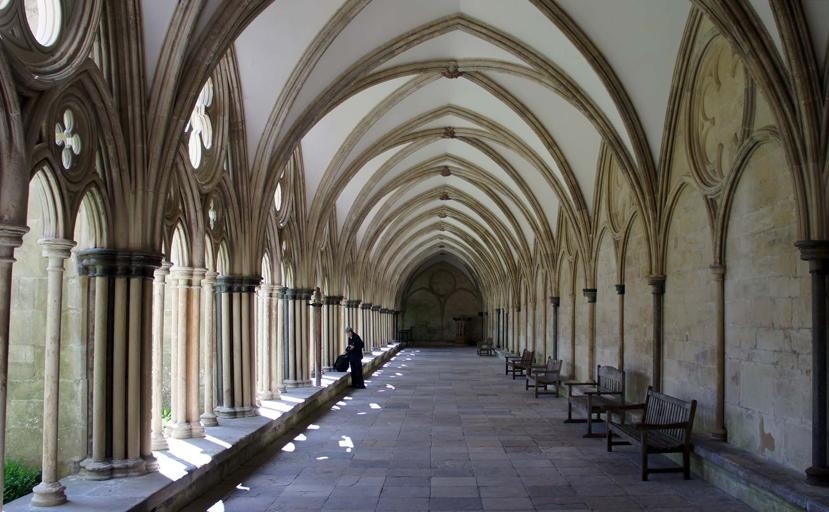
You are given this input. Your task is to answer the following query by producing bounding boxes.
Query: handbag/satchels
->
[335,354,349,372]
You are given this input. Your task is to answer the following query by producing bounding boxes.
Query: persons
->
[344,326,366,389]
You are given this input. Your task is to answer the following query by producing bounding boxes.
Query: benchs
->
[505,347,535,379]
[564,363,628,438]
[604,385,701,483]
[525,355,565,398]
[477,337,494,355]
